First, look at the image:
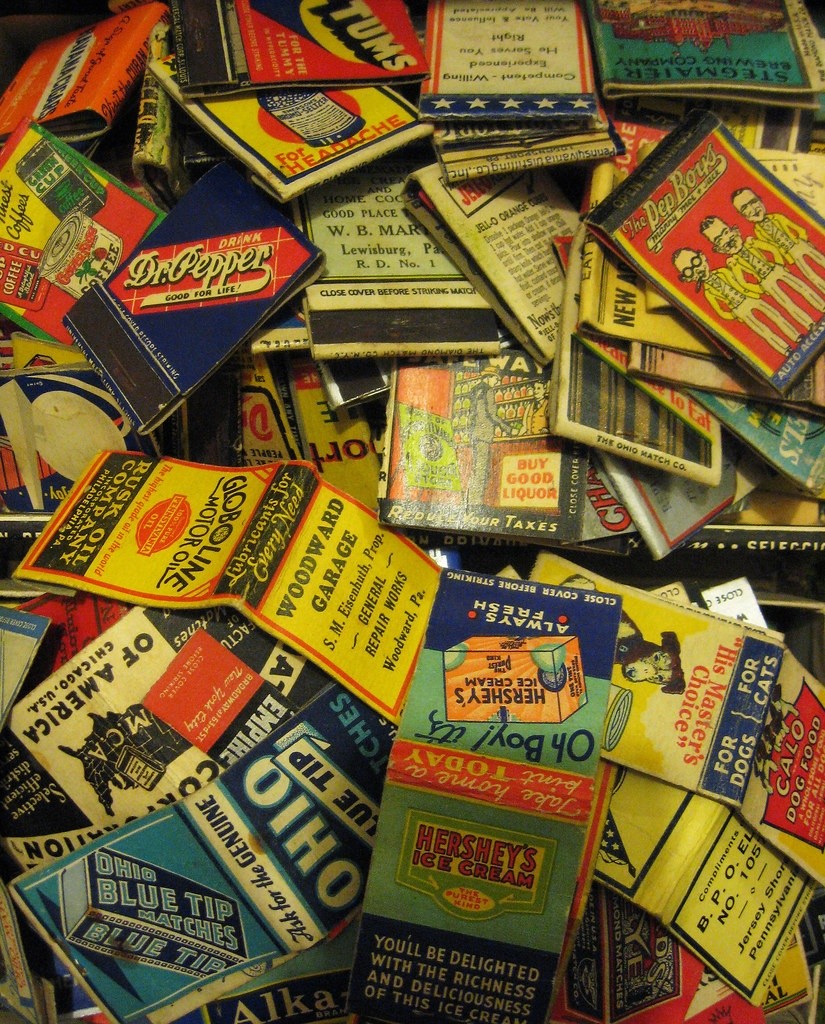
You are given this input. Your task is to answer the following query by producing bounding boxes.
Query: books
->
[0,0,825,1024]
